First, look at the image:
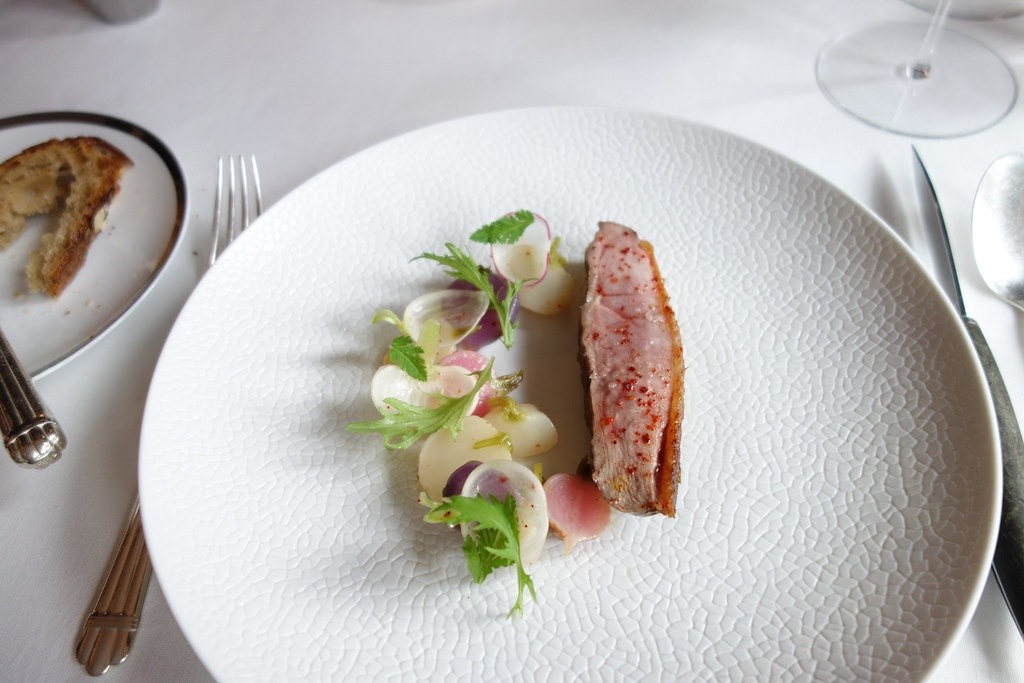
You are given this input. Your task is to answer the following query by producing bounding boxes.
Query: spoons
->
[972,152,1024,317]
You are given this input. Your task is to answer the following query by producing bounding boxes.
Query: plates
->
[0,110,190,382]
[139,105,1004,683]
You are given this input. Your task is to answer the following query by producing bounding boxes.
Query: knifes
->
[909,141,1024,641]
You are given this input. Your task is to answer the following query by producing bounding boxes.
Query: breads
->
[0,136,135,298]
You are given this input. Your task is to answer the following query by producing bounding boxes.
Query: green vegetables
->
[344,210,563,621]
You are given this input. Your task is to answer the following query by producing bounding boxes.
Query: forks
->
[74,153,261,678]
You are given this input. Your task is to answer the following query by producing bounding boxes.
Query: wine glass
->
[815,0,1018,138]
